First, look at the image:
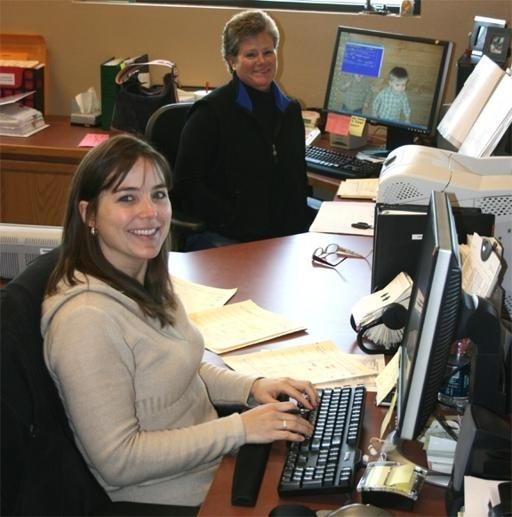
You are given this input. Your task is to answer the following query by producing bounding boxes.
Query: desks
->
[120,109,460,516]
[1,109,119,226]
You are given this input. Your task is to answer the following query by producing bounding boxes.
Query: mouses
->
[269,504,317,517]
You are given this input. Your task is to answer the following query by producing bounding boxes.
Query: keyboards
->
[304,145,378,178]
[278,385,366,494]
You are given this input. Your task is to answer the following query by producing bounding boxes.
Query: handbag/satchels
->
[111,81,179,134]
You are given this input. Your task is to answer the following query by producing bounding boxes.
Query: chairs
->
[143,103,323,244]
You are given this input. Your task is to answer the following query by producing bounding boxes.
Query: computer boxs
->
[378,144,512,319]
[457,49,512,156]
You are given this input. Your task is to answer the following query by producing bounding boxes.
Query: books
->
[337,176,381,200]
[436,54,511,158]
[186,299,307,355]
[458,231,503,301]
[1,53,151,139]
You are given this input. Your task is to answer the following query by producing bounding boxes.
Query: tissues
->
[67,85,102,129]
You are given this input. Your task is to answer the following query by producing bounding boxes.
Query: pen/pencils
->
[284,408,310,417]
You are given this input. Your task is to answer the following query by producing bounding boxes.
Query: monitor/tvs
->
[322,27,453,164]
[397,191,505,452]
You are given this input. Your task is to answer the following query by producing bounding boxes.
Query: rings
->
[282,420,287,428]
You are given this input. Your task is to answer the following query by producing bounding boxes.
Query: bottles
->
[435,333,473,416]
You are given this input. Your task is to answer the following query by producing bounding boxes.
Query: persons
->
[338,73,372,113]
[172,9,311,251]
[372,67,413,124]
[38,134,318,510]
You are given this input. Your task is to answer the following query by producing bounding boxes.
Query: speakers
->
[445,403,512,517]
[329,124,369,150]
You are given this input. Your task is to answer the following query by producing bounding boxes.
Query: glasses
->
[313,244,370,266]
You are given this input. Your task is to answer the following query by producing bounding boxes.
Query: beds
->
[0,241,198,513]
